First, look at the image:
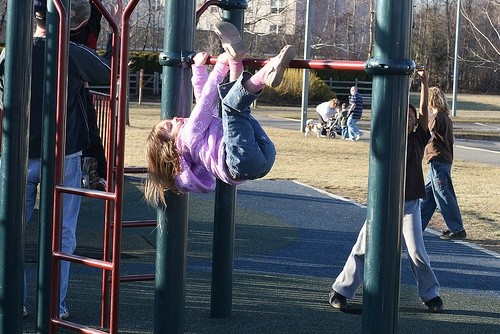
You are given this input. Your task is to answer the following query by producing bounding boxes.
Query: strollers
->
[324,110,349,138]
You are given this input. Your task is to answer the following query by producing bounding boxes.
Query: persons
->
[0,0,111,319]
[329,63,444,312]
[419,87,466,241]
[315,86,363,141]
[145,21,297,236]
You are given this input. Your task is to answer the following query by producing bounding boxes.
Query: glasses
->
[408,116,416,120]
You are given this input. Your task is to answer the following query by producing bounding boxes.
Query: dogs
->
[304,118,327,139]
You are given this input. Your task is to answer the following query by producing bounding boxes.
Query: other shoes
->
[328,290,348,311]
[423,296,445,310]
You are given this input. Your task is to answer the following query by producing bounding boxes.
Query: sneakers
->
[59,307,70,320]
[81,156,108,192]
[23,305,28,317]
[356,132,364,140]
[263,44,296,89]
[213,21,246,60]
[440,229,467,240]
[347,137,356,141]
